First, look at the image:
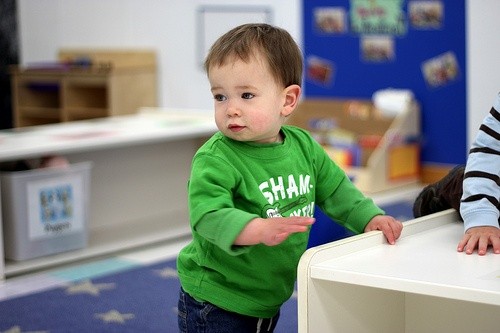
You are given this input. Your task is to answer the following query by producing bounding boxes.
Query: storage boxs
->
[0,161,94,263]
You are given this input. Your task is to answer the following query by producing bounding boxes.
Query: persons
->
[411,91,500,256]
[173,23,404,333]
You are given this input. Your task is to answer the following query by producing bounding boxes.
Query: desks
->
[297,208,500,332]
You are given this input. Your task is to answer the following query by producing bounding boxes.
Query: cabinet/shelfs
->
[12,49,160,126]
